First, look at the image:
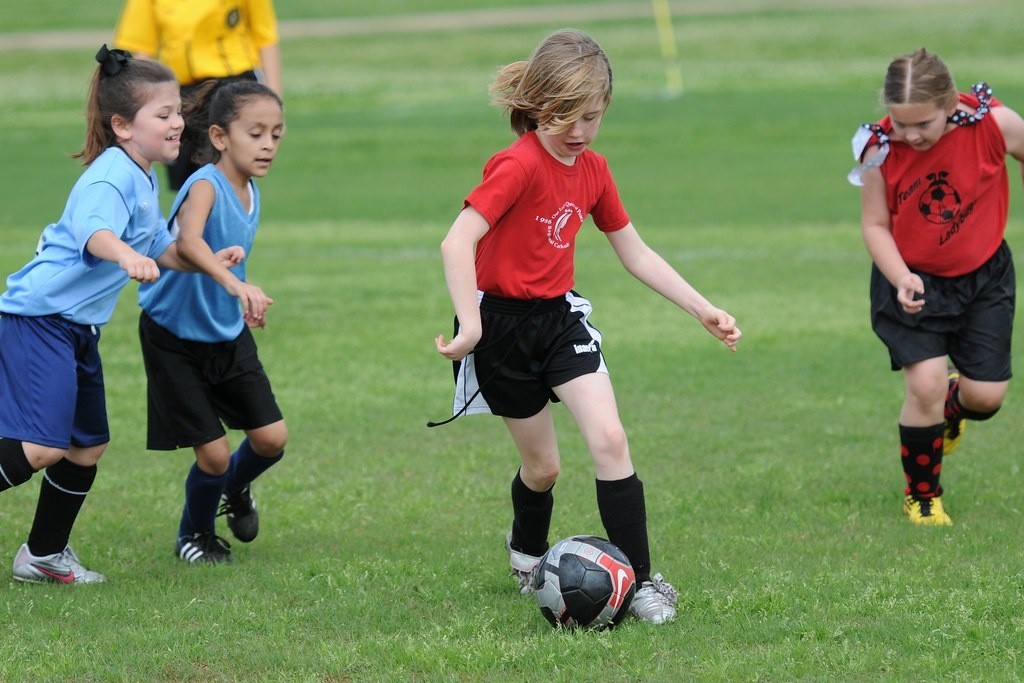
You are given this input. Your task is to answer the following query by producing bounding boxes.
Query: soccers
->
[534,534,637,632]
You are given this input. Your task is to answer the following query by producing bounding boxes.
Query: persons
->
[0,43,245,587]
[136,79,289,568]
[846,46,1024,526]
[110,0,282,192]
[434,29,743,625]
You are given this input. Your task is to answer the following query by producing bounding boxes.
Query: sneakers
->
[175,532,232,569]
[505,530,549,599]
[628,573,679,626]
[217,485,260,542]
[902,495,953,527]
[943,373,965,455]
[13,545,106,587]
